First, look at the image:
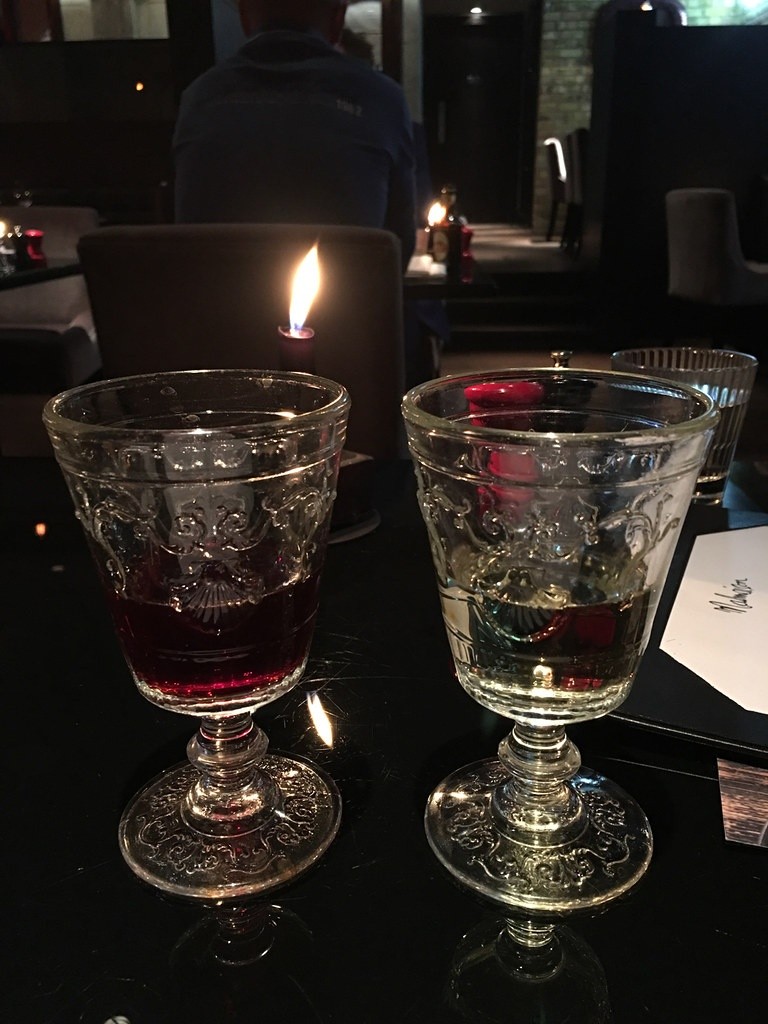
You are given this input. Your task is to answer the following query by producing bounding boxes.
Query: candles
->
[469,382,538,524]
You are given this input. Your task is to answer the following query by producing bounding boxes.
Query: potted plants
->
[277,242,326,374]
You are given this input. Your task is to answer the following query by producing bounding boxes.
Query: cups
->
[614,342,756,504]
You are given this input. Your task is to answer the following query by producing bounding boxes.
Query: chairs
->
[74,224,404,443]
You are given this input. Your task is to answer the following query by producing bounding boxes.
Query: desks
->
[406,224,568,344]
[0,457,768,1024]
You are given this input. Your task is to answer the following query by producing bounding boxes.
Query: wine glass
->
[404,370,718,911]
[44,369,351,901]
